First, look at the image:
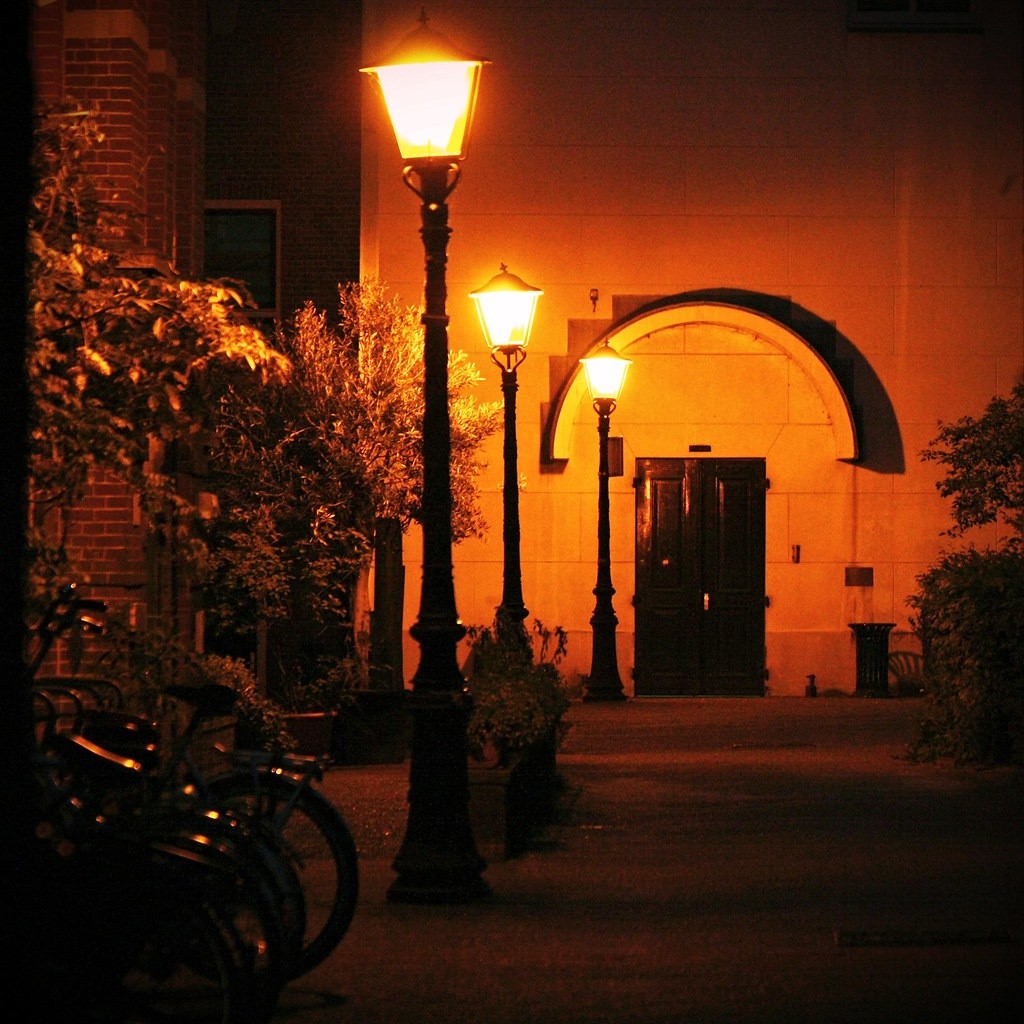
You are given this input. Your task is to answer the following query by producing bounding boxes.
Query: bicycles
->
[36,579,363,1023]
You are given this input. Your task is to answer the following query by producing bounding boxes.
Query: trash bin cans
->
[848,623,898,697]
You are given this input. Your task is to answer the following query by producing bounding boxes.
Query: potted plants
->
[465,671,556,859]
[281,663,338,754]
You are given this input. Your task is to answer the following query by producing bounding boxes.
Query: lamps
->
[590,287,598,310]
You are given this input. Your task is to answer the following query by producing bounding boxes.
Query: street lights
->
[464,263,543,658]
[353,8,497,915]
[580,334,632,703]
